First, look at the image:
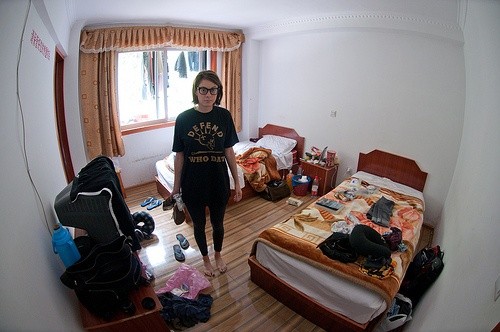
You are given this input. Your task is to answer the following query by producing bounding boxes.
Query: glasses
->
[197,87,219,95]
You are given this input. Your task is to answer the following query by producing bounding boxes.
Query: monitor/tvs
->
[54,157,140,250]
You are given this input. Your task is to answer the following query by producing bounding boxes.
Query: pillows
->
[257,134,298,157]
[232,140,272,163]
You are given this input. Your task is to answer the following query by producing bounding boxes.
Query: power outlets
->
[346,167,350,175]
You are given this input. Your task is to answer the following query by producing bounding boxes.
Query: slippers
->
[146,200,162,210]
[141,197,157,207]
[176,233,189,249]
[173,244,185,261]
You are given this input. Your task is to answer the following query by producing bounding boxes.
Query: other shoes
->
[163,193,186,225]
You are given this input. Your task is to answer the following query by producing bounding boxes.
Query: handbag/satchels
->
[373,293,413,332]
[260,179,291,202]
[383,227,402,251]
[165,263,212,301]
[318,232,359,263]
[413,245,445,291]
[59,234,147,322]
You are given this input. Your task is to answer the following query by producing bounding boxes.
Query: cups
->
[325,150,336,166]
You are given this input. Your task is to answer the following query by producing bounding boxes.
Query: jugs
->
[50,223,79,267]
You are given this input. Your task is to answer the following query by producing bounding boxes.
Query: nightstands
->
[297,157,338,195]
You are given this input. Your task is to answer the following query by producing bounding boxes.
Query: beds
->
[245,150,428,332]
[154,124,305,223]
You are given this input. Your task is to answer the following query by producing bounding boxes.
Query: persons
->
[170,71,242,277]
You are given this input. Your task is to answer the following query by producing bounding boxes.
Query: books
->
[315,197,344,215]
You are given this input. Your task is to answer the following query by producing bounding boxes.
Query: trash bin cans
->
[292,175,311,196]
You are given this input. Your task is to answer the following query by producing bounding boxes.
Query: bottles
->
[311,176,319,196]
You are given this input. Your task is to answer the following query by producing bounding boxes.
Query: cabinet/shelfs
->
[79,228,170,332]
